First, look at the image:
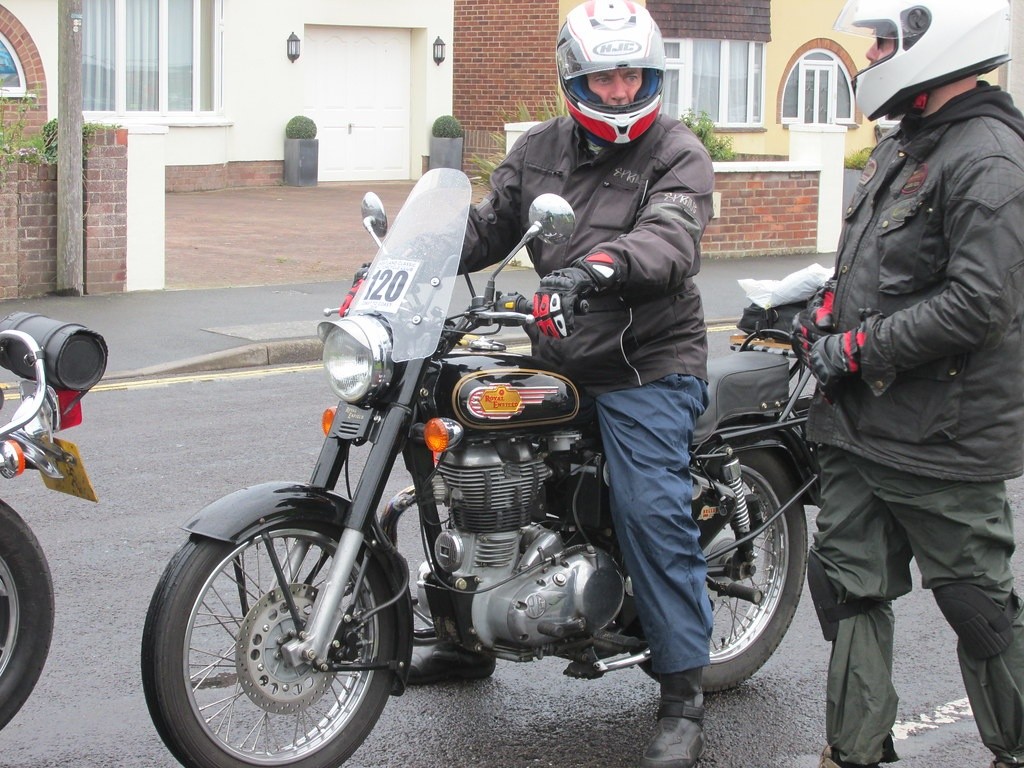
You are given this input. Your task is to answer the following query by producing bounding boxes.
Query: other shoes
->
[821,746,883,768]
[991,758,1010,768]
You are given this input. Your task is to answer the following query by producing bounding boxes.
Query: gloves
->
[533,266,595,341]
[804,326,864,395]
[788,289,837,363]
[340,265,369,315]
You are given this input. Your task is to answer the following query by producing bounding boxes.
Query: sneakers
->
[408,653,494,685]
[641,690,706,768]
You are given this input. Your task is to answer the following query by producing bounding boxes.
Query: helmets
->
[555,0,665,150]
[850,0,1013,121]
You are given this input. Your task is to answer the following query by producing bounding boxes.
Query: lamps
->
[287,32,303,62]
[434,37,447,67]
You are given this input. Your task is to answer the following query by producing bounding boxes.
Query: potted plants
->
[428,115,465,174]
[284,116,321,187]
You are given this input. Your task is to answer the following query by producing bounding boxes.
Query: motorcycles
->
[0,329,56,731]
[142,168,823,768]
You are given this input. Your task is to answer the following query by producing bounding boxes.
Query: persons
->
[341,0,713,768]
[790,0,1024,768]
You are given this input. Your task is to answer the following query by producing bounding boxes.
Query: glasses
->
[872,23,895,49]
